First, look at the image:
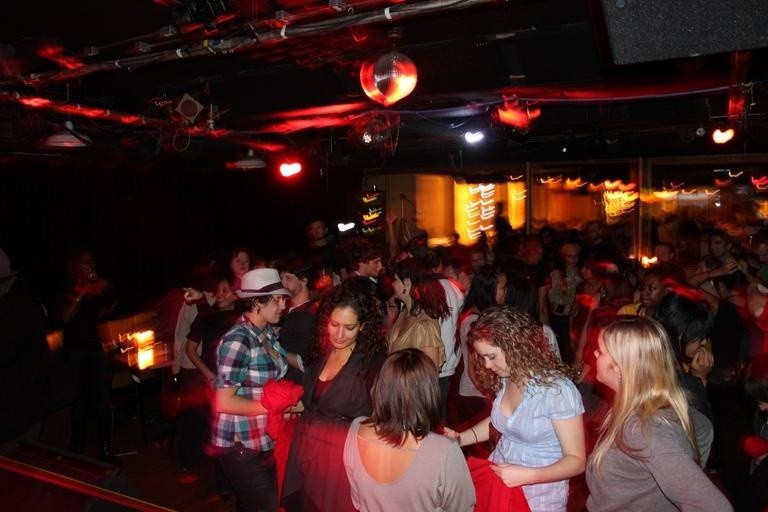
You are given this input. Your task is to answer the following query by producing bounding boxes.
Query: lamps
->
[233,147,266,169]
[464,112,507,146]
[46,78,92,148]
[359,116,392,145]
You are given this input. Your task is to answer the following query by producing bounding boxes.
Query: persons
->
[2,203,767,511]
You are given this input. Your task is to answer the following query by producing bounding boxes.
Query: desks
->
[104,366,167,457]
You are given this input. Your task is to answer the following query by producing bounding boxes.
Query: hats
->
[235,268,293,298]
